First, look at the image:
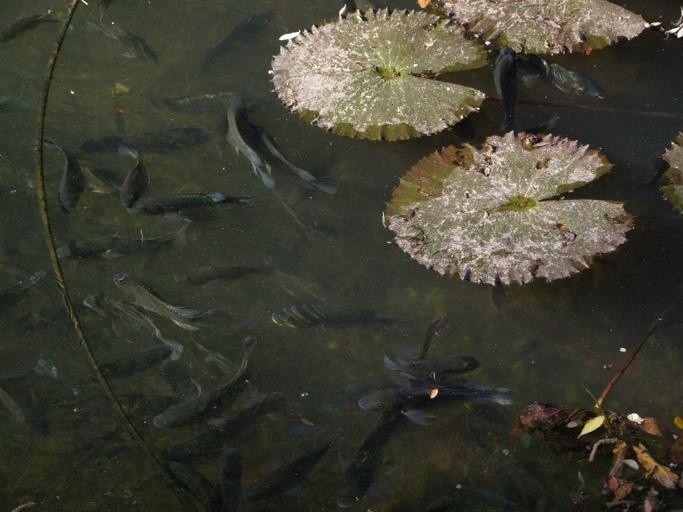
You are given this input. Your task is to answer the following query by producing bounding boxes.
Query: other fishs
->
[534,55,603,100]
[1,1,519,510]
